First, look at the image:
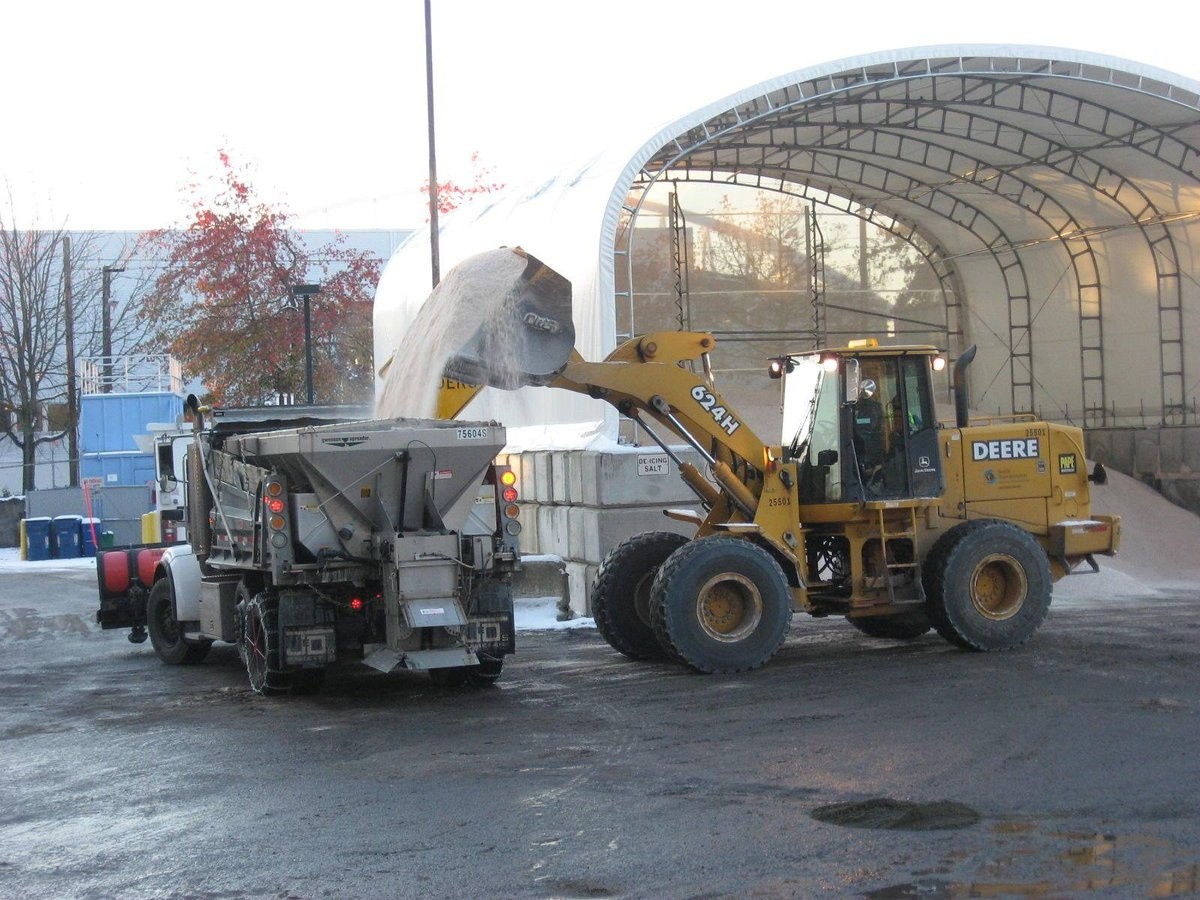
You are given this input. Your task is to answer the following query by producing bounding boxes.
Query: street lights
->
[293,283,322,404]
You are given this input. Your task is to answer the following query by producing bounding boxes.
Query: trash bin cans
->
[54,515,82,559]
[82,518,102,557]
[25,516,52,561]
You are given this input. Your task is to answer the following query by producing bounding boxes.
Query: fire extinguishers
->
[164,520,176,541]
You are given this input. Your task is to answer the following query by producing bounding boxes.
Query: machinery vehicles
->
[378,241,1125,677]
[94,400,521,695]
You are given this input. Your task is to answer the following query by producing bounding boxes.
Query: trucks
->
[75,394,185,515]
